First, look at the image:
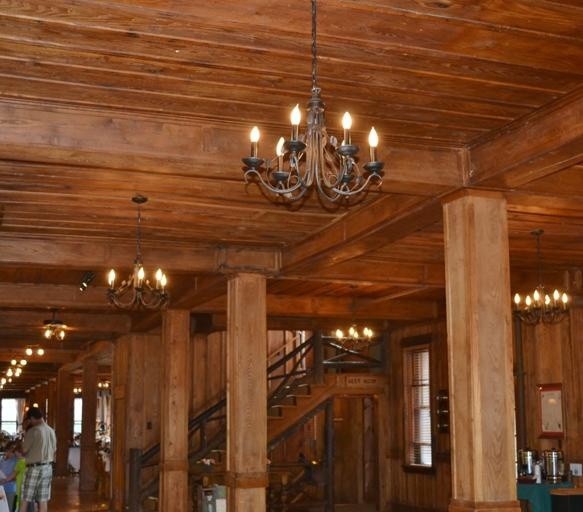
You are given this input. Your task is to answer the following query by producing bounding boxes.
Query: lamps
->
[242,0,384,203]
[106,196,167,312]
[335,284,374,352]
[0,308,65,388]
[513,228,568,325]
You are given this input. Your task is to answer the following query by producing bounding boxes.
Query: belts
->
[25,461,54,466]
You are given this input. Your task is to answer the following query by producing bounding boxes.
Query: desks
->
[517,481,583,512]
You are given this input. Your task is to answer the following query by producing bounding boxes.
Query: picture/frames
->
[535,383,565,440]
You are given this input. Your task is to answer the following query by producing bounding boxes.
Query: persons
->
[0,408,57,511]
